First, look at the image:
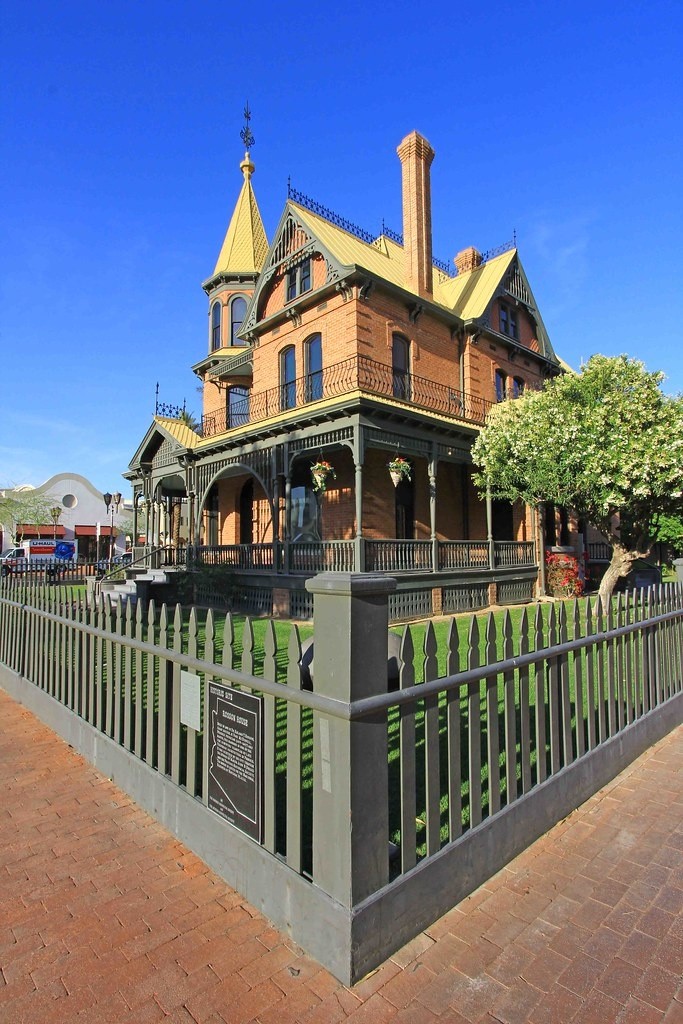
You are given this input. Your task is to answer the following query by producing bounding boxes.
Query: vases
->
[312,469,331,489]
[389,468,405,488]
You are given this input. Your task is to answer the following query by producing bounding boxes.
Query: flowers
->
[310,461,337,492]
[387,457,412,483]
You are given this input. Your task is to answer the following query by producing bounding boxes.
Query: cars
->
[94,551,132,574]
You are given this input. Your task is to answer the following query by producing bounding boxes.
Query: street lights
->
[103,491,122,572]
[50,507,62,540]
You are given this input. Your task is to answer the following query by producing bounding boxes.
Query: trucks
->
[0,539,78,578]
